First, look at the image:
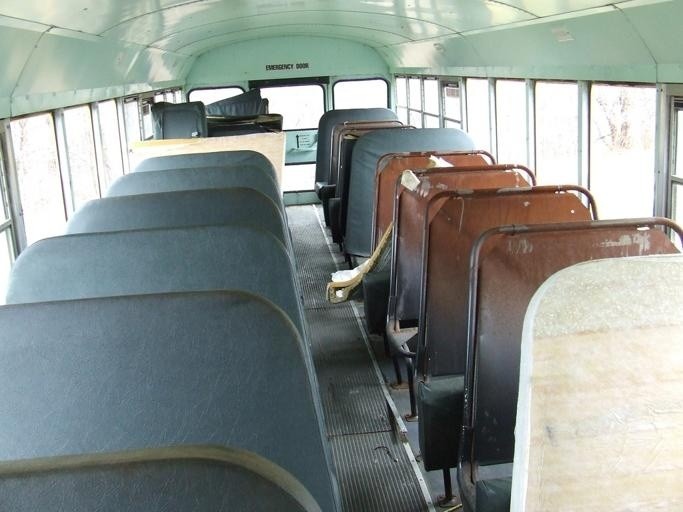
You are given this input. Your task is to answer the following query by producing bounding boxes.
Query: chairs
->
[310,87,683,511]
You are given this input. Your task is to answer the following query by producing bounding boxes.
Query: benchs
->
[2,88,345,511]
